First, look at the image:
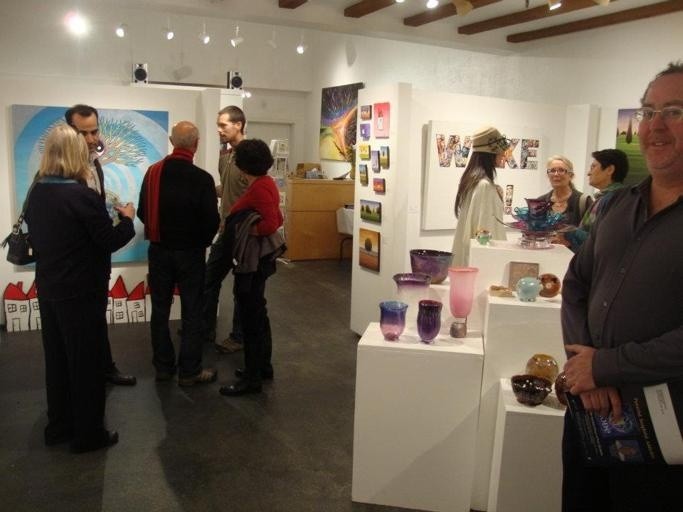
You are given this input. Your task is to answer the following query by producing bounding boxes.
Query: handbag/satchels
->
[5,232,36,265]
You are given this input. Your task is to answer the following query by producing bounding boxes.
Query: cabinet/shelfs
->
[282,178,356,261]
[349,231,570,512]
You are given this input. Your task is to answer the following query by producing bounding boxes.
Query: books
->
[563,381,682,473]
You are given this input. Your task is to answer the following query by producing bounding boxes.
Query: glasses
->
[636,106,682,119]
[546,168,567,176]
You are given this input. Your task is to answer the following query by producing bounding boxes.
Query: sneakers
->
[220,337,244,354]
[179,368,217,386]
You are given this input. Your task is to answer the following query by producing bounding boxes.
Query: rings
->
[560,373,567,381]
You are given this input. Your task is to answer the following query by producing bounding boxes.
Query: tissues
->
[307,168,319,179]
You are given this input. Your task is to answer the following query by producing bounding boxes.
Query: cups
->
[379,266,478,342]
[525,197,555,222]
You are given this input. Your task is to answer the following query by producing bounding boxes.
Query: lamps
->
[108,8,315,57]
[394,1,612,18]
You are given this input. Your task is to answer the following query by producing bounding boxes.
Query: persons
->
[558,148,630,253]
[21,123,136,453]
[536,154,593,227]
[176,105,245,354]
[64,103,137,386]
[559,59,683,511]
[218,136,284,397]
[136,119,221,389]
[450,127,510,267]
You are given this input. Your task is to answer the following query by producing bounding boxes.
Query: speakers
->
[227,72,243,89]
[132,64,148,84]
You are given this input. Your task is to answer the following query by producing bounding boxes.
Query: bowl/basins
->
[409,249,454,284]
[512,375,551,407]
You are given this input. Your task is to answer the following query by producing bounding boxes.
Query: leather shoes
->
[110,372,136,386]
[219,380,262,396]
[235,368,273,381]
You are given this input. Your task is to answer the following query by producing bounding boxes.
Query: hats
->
[472,127,508,153]
